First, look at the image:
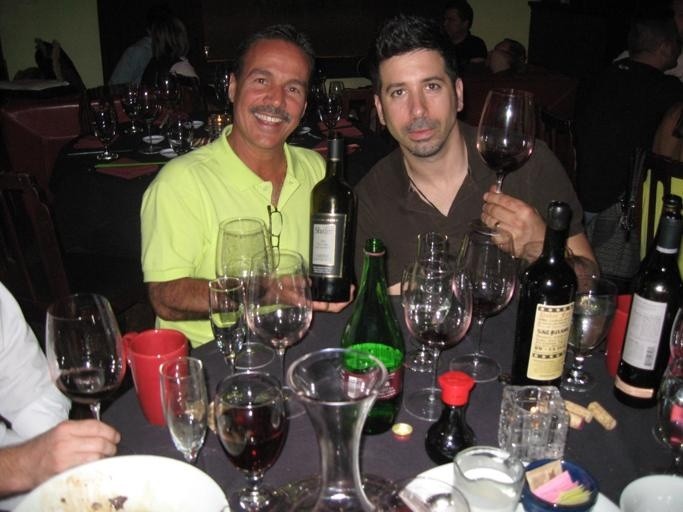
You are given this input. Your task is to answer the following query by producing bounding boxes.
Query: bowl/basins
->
[519,456,600,511]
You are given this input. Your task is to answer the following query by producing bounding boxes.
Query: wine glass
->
[157,356,211,460]
[40,292,128,459]
[214,374,292,509]
[516,235,606,396]
[86,50,346,157]
[654,307,682,475]
[202,221,313,422]
[568,284,606,392]
[402,228,510,430]
[477,83,539,199]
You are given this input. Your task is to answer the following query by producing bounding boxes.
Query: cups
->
[123,328,192,423]
[449,442,526,508]
[497,382,568,456]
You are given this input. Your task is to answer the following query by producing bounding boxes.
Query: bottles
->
[510,203,578,384]
[424,368,478,463]
[615,190,680,405]
[268,347,421,512]
[304,136,359,305]
[343,237,401,437]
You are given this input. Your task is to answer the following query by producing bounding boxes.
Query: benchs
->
[0,99,188,204]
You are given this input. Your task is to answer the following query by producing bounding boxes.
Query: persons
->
[335,20,603,314]
[11,29,88,94]
[610,2,681,80]
[577,14,682,231]
[110,8,152,84]
[649,98,682,166]
[467,37,527,88]
[140,19,193,88]
[1,274,118,498]
[432,1,489,69]
[142,29,350,347]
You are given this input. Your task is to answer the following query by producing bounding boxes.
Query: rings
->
[494,219,500,232]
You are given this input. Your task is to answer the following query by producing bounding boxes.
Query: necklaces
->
[405,166,446,220]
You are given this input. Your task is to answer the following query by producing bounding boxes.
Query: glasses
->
[265,204,283,269]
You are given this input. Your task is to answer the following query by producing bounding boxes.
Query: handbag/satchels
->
[591,146,648,278]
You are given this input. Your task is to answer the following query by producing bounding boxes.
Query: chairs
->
[1,171,147,353]
[533,102,578,189]
[339,87,376,134]
[626,145,683,263]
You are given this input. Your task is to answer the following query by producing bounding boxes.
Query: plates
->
[393,458,615,512]
[9,453,230,511]
[617,469,683,512]
